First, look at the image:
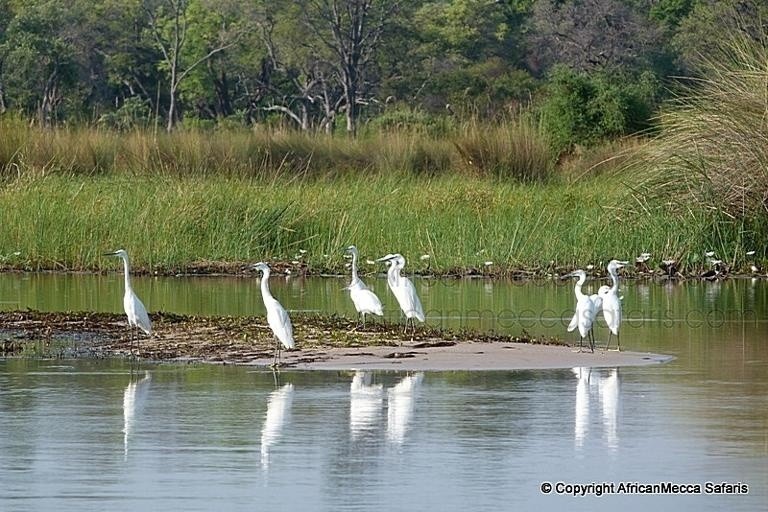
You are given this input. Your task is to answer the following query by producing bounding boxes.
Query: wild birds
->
[375,252,427,334]
[559,267,600,354]
[247,260,297,367]
[100,248,155,353]
[597,257,630,350]
[341,245,385,331]
